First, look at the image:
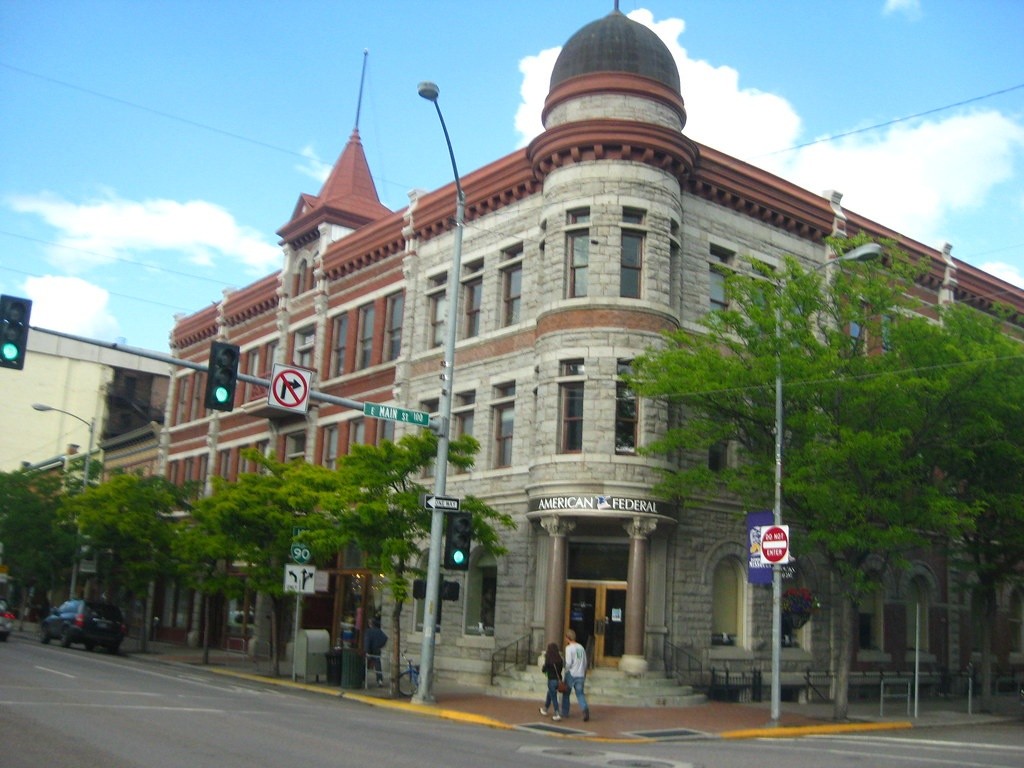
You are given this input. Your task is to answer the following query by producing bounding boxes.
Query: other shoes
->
[537,707,544,716]
[550,715,561,721]
[582,706,590,721]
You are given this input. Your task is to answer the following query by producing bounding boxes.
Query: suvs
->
[40,599,130,654]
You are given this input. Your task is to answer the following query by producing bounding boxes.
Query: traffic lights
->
[0,292,33,372]
[204,340,241,412]
[444,511,473,570]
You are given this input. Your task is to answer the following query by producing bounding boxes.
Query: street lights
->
[410,77,466,703]
[770,243,884,727]
[31,401,96,598]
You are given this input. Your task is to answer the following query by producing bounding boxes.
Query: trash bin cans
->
[295,628,330,683]
[341,652,363,688]
[324,652,341,686]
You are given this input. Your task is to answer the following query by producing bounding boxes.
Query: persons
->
[560,630,591,723]
[364,615,388,687]
[538,643,565,720]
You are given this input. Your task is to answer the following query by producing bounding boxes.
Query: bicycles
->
[398,648,419,697]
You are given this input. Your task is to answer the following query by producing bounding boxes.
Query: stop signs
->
[760,524,790,566]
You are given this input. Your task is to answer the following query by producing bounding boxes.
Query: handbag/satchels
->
[557,682,567,694]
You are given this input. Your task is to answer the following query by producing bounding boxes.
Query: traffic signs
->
[424,494,461,513]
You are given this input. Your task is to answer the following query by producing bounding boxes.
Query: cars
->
[0,595,16,642]
